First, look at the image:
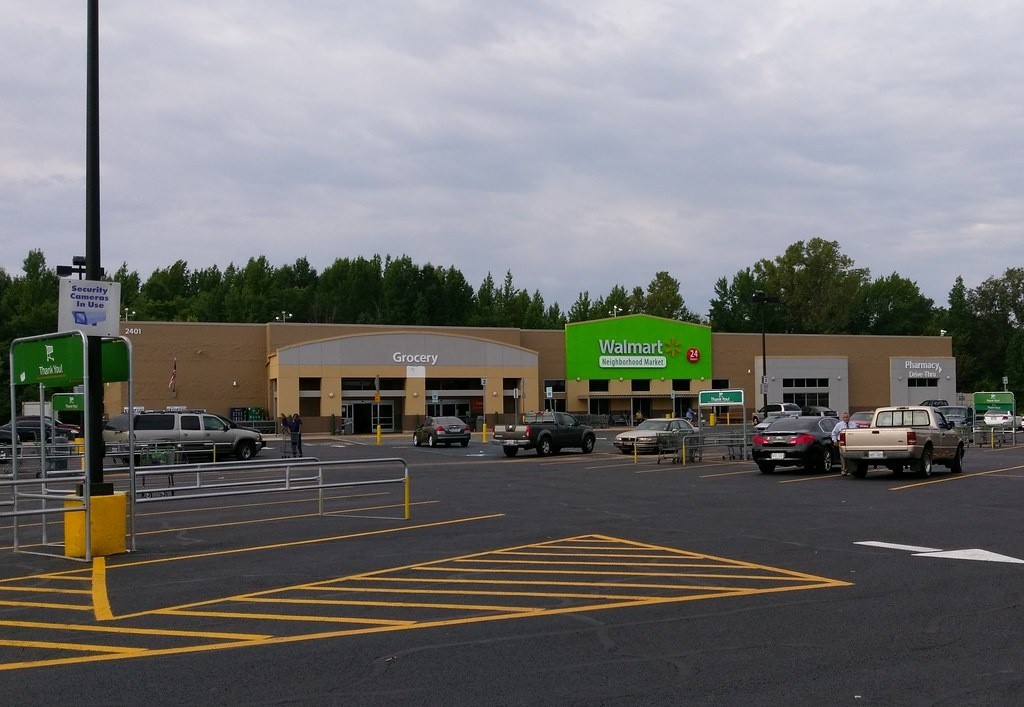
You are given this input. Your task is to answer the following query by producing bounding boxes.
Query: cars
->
[752,416,840,473]
[0,430,21,463]
[937,406,974,442]
[9,415,81,433]
[849,410,885,428]
[753,415,782,434]
[213,412,263,442]
[970,403,1024,430]
[613,418,703,454]
[803,406,839,421]
[413,416,470,447]
[1,420,80,443]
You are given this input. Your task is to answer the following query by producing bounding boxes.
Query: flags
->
[168,358,179,392]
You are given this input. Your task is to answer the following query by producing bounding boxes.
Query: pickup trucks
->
[491,412,596,456]
[101,409,263,465]
[920,400,948,406]
[839,406,965,479]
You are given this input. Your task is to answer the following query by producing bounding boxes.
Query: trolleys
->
[281,432,301,458]
[34,441,77,478]
[0,442,26,474]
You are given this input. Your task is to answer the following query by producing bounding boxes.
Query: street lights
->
[57,255,104,279]
[275,310,293,323]
[120,308,136,322]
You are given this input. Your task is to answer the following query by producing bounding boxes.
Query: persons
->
[280,411,290,437]
[831,412,859,477]
[622,410,632,426]
[288,414,303,458]
[686,405,698,424]
[635,409,644,426]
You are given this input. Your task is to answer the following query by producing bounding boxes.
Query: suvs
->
[752,401,803,426]
